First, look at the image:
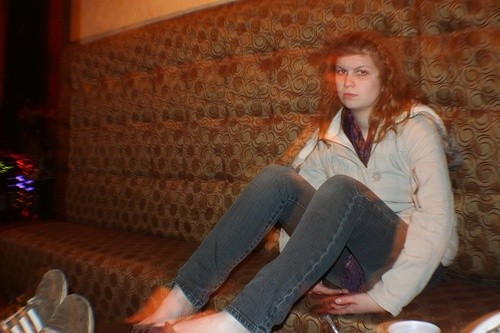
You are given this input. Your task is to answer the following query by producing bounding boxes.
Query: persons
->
[130,31,459,333]
[0,267,95,332]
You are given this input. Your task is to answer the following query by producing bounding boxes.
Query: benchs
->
[0,0,500,333]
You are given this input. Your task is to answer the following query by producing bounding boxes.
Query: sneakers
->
[0,266,67,333]
[41,293,96,333]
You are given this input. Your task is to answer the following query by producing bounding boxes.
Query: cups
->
[388,320,441,333]
